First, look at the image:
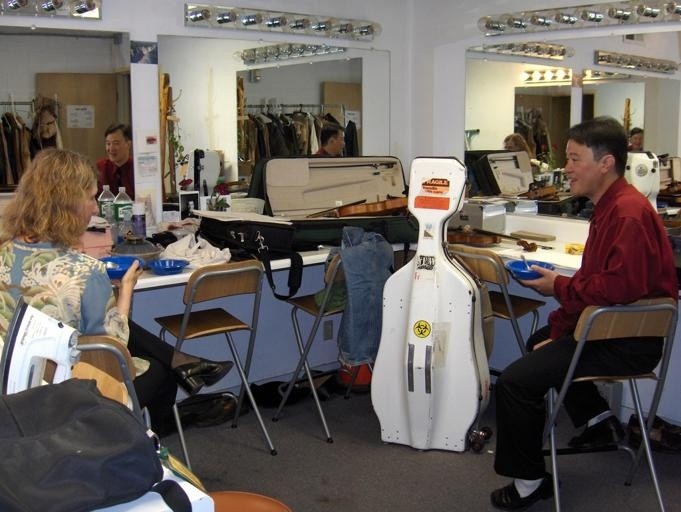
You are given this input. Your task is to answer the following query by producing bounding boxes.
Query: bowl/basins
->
[97,255,145,280]
[147,259,190,276]
[505,259,555,280]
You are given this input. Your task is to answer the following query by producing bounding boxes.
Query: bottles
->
[132,203,147,239]
[97,185,116,226]
[113,187,132,236]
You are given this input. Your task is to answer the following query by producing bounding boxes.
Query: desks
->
[96,225,679,295]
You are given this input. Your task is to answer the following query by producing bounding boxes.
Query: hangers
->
[0,102,37,120]
[245,105,334,119]
[515,106,544,127]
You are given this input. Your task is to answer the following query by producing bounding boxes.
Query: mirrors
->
[584,70,679,162]
[466,51,572,187]
[157,35,391,221]
[0,24,135,230]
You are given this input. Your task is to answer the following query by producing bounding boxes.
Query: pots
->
[110,235,163,269]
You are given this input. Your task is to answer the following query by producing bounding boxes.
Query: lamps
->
[184,3,377,42]
[477,0,681,37]
[594,51,677,74]
[0,1,101,21]
[522,68,572,84]
[242,45,345,63]
[584,70,638,81]
[471,41,564,60]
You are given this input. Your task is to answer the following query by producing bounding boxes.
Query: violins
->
[516,185,557,201]
[306,193,408,218]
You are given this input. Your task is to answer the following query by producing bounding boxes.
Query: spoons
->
[521,255,532,270]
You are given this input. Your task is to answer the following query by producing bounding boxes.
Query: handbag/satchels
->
[0,379,193,512]
[195,217,304,301]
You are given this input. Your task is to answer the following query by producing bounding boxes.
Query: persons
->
[624,127,644,153]
[91,121,167,202]
[0,147,234,453]
[504,108,552,165]
[314,123,346,158]
[489,116,678,512]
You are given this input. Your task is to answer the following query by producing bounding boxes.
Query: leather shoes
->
[489,470,560,511]
[567,414,626,446]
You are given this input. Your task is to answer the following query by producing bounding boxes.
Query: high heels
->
[172,356,233,394]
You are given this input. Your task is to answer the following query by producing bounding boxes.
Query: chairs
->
[527,297,678,511]
[441,241,548,415]
[70,335,148,431]
[153,258,276,473]
[271,241,394,443]
